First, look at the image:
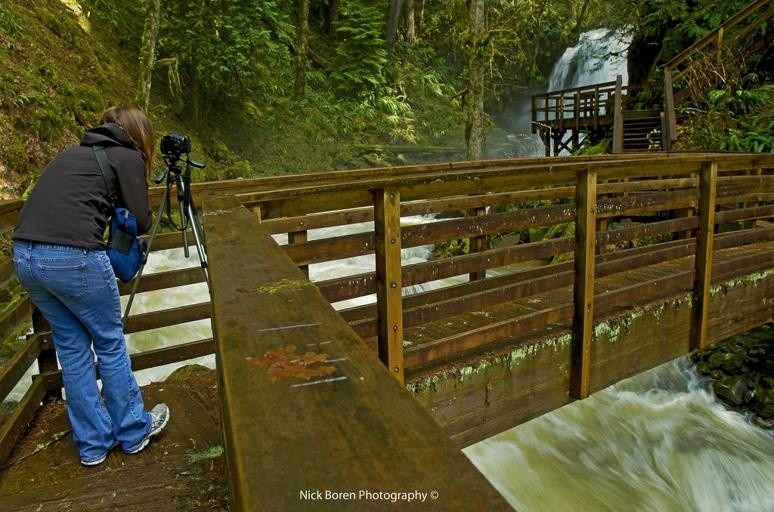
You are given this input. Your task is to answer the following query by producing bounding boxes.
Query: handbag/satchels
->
[106,208,147,283]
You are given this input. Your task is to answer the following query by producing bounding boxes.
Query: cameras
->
[160,133,192,156]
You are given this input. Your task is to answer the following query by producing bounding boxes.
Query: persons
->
[10,106,171,467]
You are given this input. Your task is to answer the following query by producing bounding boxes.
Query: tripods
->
[121,166,209,328]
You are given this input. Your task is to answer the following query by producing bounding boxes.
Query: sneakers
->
[123,403,170,454]
[80,440,119,466]
